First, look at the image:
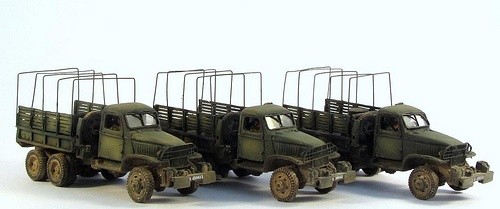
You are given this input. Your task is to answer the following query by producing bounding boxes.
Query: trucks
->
[282,66,494,200]
[15,67,216,203]
[152,69,356,202]
